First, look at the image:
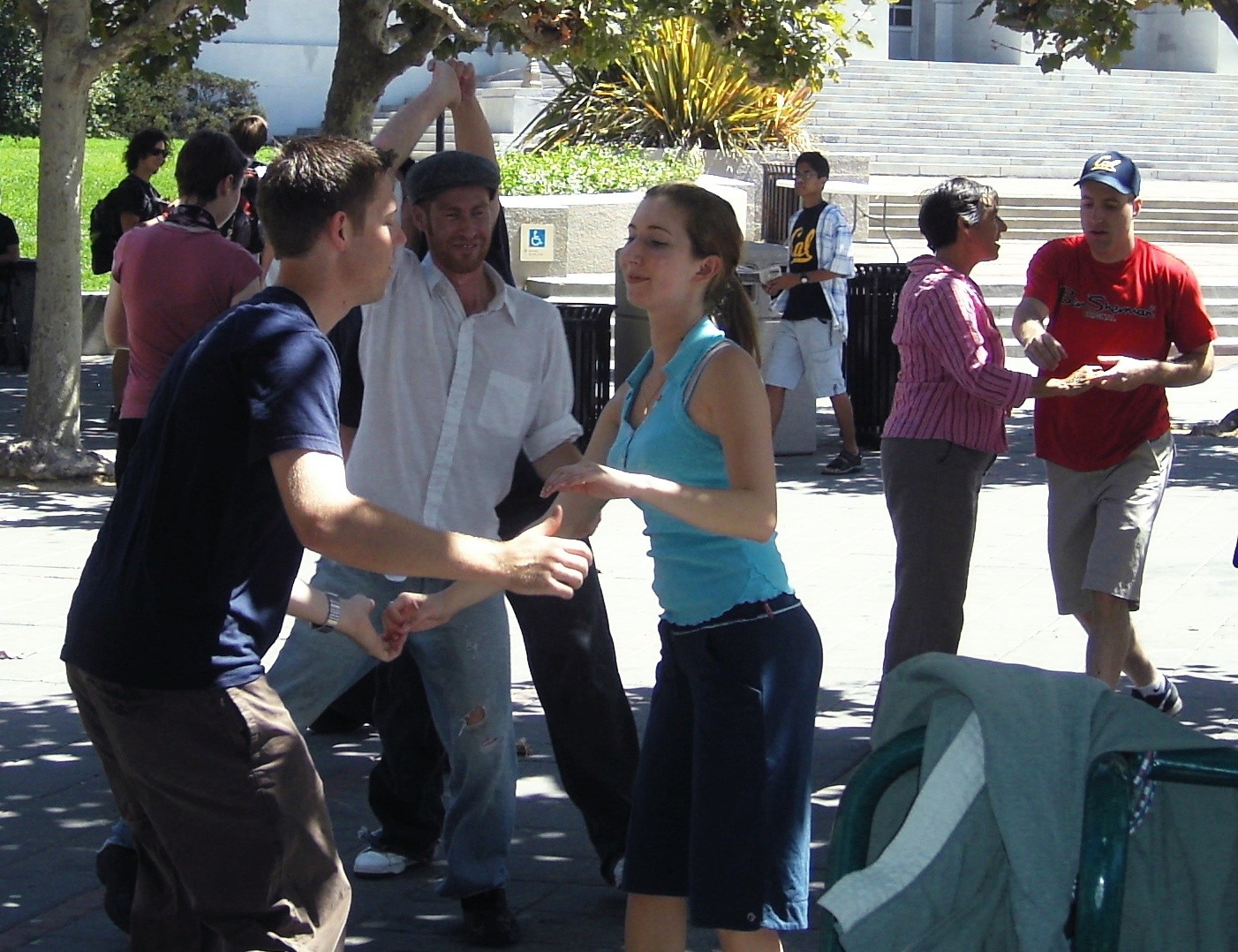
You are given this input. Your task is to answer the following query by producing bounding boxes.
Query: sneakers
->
[613,856,628,888]
[1128,676,1184,718]
[353,845,416,874]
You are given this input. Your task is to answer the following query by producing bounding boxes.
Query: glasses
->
[146,146,170,158]
[791,171,820,180]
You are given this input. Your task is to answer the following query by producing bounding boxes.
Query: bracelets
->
[311,592,341,634]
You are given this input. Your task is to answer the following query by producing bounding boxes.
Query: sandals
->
[822,450,865,473]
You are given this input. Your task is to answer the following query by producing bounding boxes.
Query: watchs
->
[800,272,809,283]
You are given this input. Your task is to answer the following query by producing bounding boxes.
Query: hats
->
[1074,150,1140,200]
[405,151,501,207]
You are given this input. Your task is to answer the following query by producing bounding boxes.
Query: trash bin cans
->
[737,242,817,457]
[549,301,617,457]
[759,162,800,246]
[842,262,910,451]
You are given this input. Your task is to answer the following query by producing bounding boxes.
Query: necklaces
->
[639,376,666,415]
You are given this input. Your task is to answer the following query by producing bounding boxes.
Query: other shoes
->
[108,405,122,431]
[305,719,373,734]
[459,887,521,946]
[97,844,140,932]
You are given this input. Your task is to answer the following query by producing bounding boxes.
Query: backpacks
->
[219,160,267,255]
[90,187,160,274]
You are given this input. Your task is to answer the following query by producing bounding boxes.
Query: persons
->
[1012,153,1219,717]
[763,151,864,475]
[0,57,826,952]
[871,177,1102,724]
